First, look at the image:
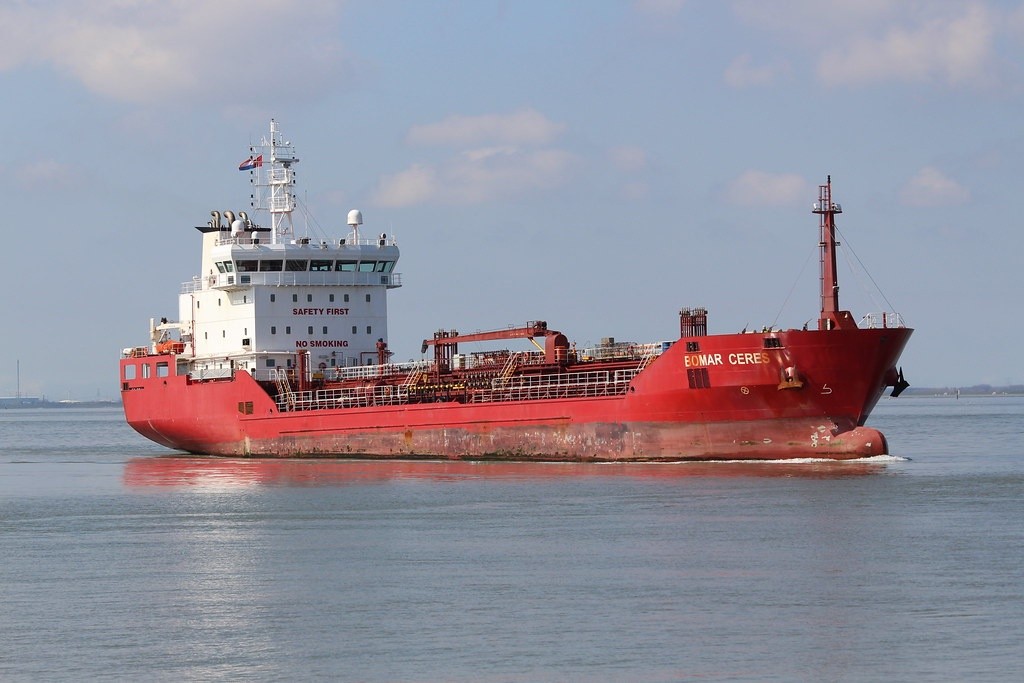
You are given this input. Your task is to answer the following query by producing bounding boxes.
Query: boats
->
[118,117,914,462]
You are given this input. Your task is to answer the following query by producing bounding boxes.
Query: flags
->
[239,154,262,171]
